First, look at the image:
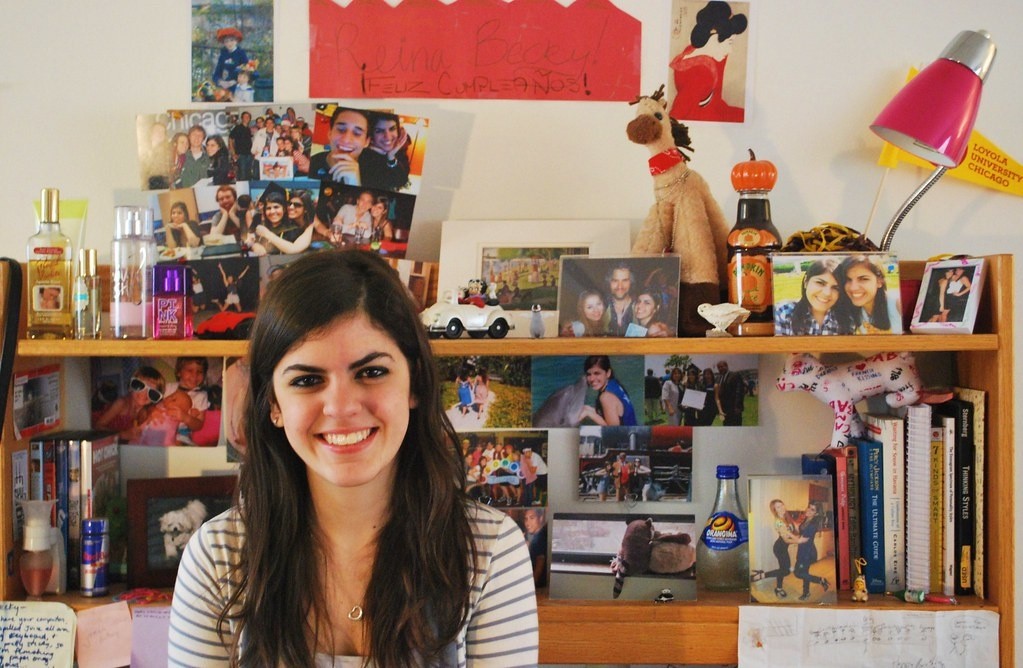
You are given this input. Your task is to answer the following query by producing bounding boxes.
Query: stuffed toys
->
[775,352,921,448]
[611,517,695,599]
[626,84,730,336]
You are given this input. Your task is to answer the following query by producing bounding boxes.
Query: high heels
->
[750,570,766,583]
[821,578,830,592]
[798,592,810,602]
[775,587,786,597]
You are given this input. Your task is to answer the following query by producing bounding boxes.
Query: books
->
[802,385,986,594]
[28,429,121,592]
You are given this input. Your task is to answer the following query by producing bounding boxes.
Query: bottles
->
[75,249,103,342]
[696,465,749,592]
[26,189,75,342]
[885,589,925,603]
[152,265,195,340]
[727,189,783,336]
[111,206,156,338]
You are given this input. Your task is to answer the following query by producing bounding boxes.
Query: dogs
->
[156,500,210,559]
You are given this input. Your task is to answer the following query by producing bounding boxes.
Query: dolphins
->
[532,376,590,428]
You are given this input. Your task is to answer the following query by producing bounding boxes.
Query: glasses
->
[620,455,625,459]
[287,201,304,208]
[635,461,641,463]
[130,378,162,404]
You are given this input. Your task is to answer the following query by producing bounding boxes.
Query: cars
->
[418,296,516,339]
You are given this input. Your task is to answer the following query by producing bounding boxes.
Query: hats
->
[686,363,701,377]
[463,439,469,443]
[620,453,625,456]
[505,445,513,449]
[259,181,288,204]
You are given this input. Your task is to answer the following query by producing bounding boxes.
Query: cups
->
[355,226,365,244]
[330,224,344,246]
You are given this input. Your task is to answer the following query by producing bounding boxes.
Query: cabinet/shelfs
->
[0,252,1023,668]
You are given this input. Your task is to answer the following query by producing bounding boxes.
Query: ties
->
[719,375,725,391]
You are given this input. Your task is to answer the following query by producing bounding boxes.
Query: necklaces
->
[348,606,364,621]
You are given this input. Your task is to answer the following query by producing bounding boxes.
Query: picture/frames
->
[909,257,988,334]
[126,475,239,591]
[437,220,631,340]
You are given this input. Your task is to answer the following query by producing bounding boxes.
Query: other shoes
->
[506,497,513,506]
[498,496,505,502]
[532,501,541,505]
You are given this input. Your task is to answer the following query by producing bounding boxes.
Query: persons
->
[929,267,972,322]
[457,279,488,308]
[310,106,410,192]
[37,288,59,309]
[168,248,539,668]
[187,263,250,313]
[144,108,312,189]
[489,257,558,297]
[645,360,755,427]
[774,255,902,336]
[579,355,637,427]
[560,263,674,337]
[752,499,832,600]
[595,452,652,502]
[452,364,546,582]
[166,181,393,255]
[98,357,250,455]
[213,27,259,103]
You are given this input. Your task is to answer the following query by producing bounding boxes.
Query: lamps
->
[868,28,997,250]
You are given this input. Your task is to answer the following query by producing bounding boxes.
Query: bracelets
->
[387,159,397,168]
